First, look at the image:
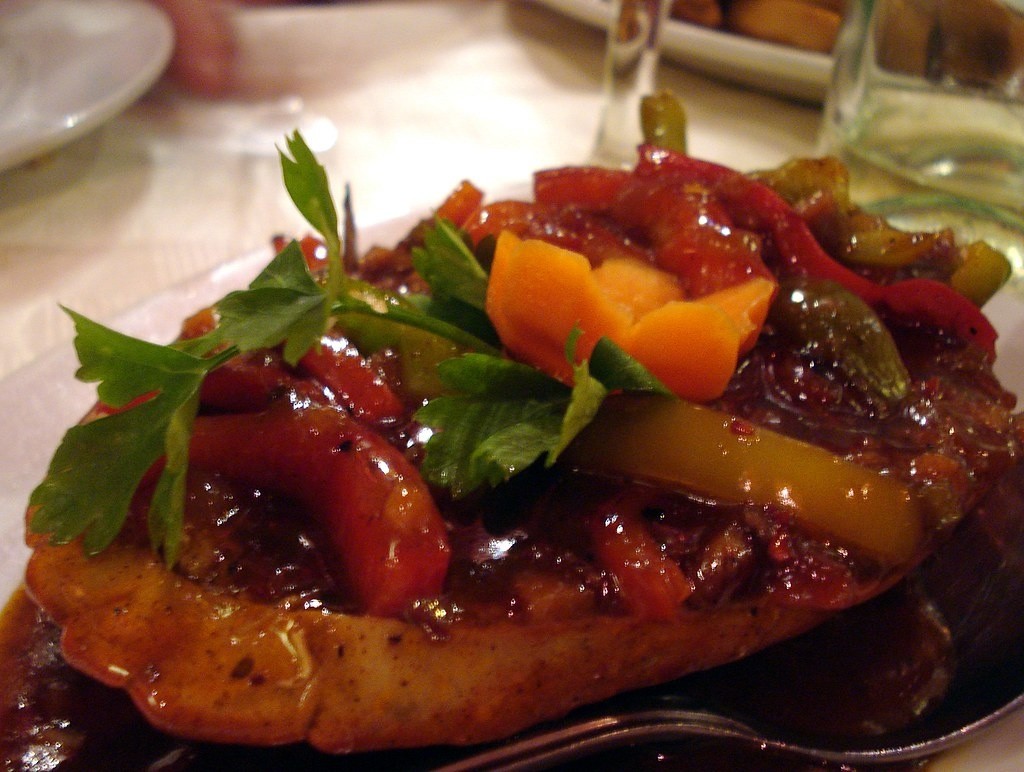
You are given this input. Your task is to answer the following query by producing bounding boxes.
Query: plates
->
[0,0,178,172]
[0,233,274,614]
[535,0,837,102]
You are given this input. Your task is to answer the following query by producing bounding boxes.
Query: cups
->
[812,1,1022,297]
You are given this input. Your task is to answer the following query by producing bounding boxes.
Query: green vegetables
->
[24,128,681,569]
